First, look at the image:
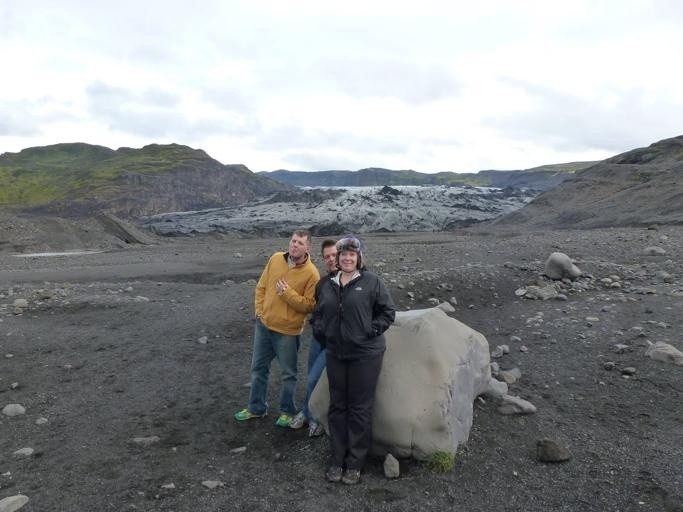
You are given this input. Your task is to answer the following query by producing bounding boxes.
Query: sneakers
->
[342,470,362,484]
[309,421,325,437]
[233,403,269,422]
[275,411,308,429]
[327,465,343,481]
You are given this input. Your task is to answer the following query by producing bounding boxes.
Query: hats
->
[335,233,362,251]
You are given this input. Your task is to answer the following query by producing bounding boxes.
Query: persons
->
[231,227,320,430]
[311,233,397,484]
[287,238,340,437]
